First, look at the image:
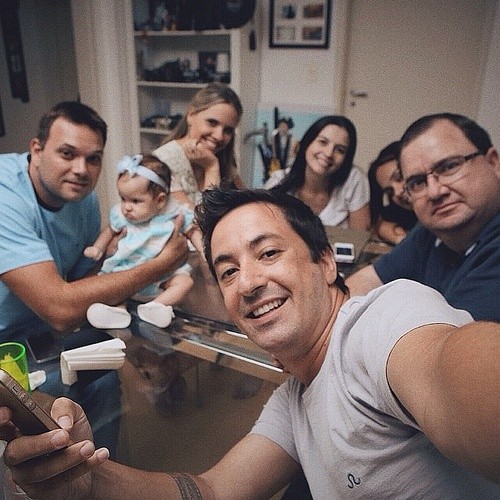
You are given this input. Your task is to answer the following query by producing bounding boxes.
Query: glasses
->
[402,150,486,199]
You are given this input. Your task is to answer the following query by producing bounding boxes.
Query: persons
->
[368,141,418,246]
[344,114,500,324]
[83,152,207,329]
[269,117,298,170]
[150,83,244,212]
[0,183,500,500]
[0,100,190,463]
[261,115,371,231]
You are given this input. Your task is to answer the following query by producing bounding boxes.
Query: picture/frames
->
[269,0,332,50]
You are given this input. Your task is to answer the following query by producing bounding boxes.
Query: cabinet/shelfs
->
[125,0,261,202]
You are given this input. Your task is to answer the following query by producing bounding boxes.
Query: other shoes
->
[137,301,175,328]
[232,373,264,399]
[156,376,186,417]
[86,303,131,329]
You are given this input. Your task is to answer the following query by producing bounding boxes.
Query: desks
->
[114,226,410,388]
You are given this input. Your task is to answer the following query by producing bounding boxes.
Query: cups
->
[0,342,32,397]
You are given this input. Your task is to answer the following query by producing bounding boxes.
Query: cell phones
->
[0,368,74,450]
[334,241,355,263]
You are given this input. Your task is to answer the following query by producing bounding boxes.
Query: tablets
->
[24,324,115,365]
